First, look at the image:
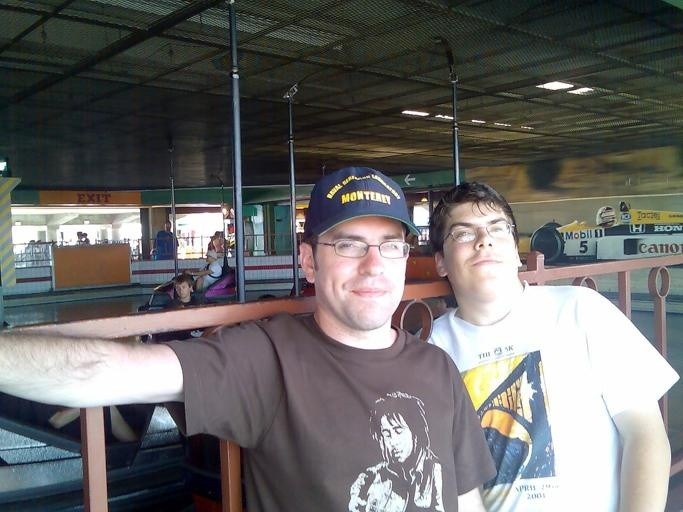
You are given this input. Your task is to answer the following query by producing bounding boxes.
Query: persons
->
[156,274,202,341]
[153,220,179,260]
[81,231,91,244]
[412,181,681,512]
[206,231,224,253]
[0,164,497,512]
[184,250,223,291]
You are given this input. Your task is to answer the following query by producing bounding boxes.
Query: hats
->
[306,168,424,239]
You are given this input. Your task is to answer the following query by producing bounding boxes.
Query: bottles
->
[151,249,156,260]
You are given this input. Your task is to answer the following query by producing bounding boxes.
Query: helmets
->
[206,251,217,259]
[597,207,615,226]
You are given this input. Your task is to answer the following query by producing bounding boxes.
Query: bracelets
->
[192,272,194,276]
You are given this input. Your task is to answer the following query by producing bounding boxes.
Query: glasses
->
[444,223,519,243]
[311,241,410,259]
